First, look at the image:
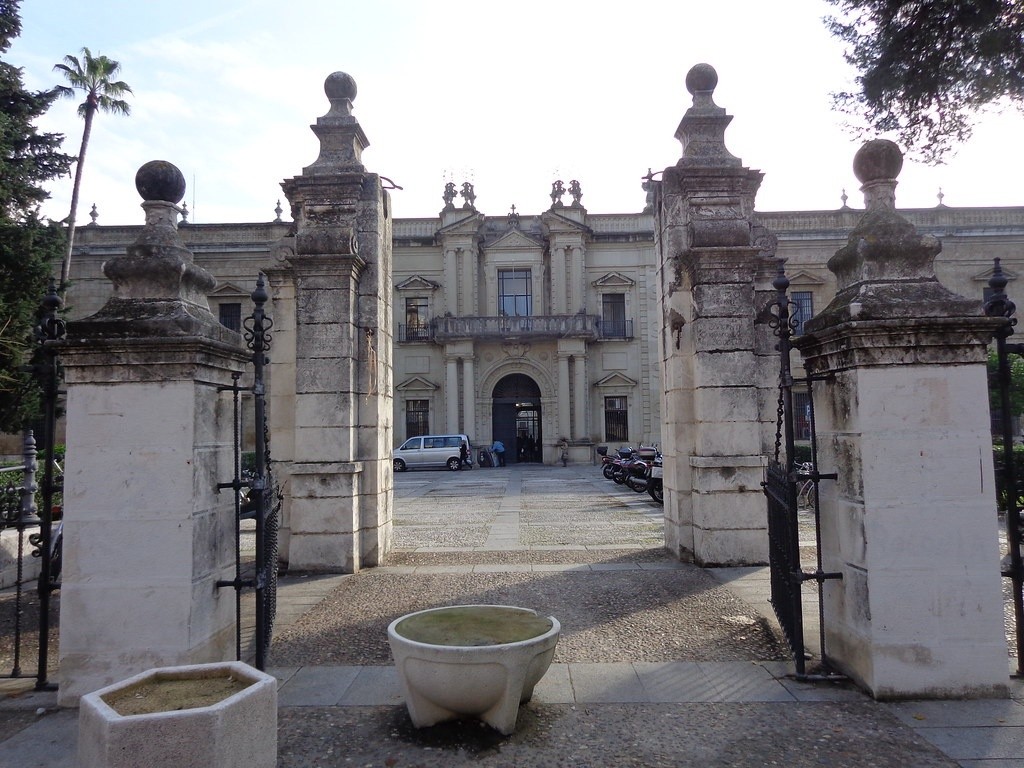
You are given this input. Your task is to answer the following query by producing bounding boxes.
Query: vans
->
[392,434,470,471]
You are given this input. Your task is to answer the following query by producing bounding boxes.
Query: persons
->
[489,440,506,467]
[515,430,542,463]
[559,439,568,467]
[457,440,473,470]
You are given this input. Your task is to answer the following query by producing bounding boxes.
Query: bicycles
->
[792,459,814,510]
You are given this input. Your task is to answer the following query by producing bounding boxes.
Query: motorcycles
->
[596,441,663,504]
